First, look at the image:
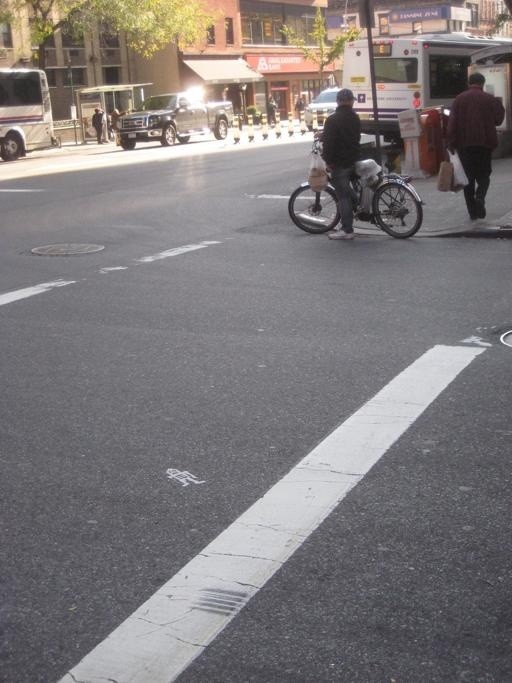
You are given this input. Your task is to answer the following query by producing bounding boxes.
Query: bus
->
[340,31,511,147]
[0,68,56,161]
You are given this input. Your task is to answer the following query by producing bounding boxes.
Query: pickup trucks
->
[115,92,234,148]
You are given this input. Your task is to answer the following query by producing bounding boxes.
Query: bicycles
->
[289,136,422,237]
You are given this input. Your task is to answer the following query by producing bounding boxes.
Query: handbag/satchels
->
[309,152,329,192]
[438,149,469,193]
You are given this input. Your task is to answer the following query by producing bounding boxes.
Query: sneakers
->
[328,230,354,239]
[466,199,486,220]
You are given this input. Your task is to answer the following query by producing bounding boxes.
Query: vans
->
[304,87,342,130]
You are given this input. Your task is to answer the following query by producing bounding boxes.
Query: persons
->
[91,107,105,144]
[447,70,507,221]
[313,87,363,239]
[295,97,309,121]
[111,108,128,145]
[106,115,115,139]
[266,95,278,125]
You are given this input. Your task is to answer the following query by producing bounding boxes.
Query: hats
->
[354,159,382,179]
[337,88,357,100]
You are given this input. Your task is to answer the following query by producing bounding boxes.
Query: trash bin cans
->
[386,143,400,173]
